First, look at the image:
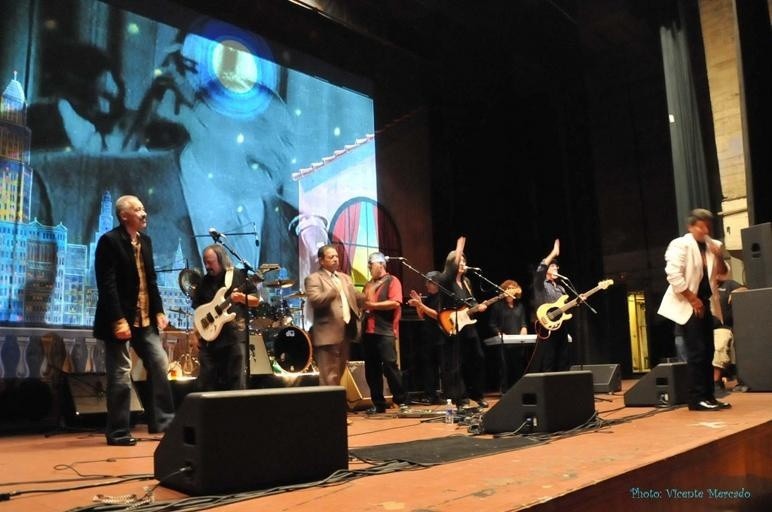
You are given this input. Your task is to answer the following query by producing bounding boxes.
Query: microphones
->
[553,272,568,281]
[704,234,717,249]
[463,266,481,273]
[208,228,220,237]
[385,256,407,262]
[253,223,259,246]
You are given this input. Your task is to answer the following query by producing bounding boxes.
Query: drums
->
[271,325,313,375]
[249,300,293,329]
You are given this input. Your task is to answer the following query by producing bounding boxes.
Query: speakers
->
[569,363,622,392]
[482,370,594,435]
[153,386,349,497]
[624,362,689,406]
[62,372,145,427]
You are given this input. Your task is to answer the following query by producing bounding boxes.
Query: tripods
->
[421,300,483,424]
[579,301,613,404]
[169,300,201,376]
[483,296,514,398]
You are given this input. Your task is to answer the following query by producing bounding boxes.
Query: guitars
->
[437,289,516,337]
[537,279,614,330]
[194,264,280,342]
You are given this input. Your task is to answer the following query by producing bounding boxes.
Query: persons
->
[710,277,748,394]
[531,238,588,371]
[490,280,529,397]
[29,77,353,291]
[191,244,259,393]
[656,207,731,411]
[404,270,443,402]
[92,193,176,447]
[361,251,407,414]
[439,234,489,410]
[305,244,359,386]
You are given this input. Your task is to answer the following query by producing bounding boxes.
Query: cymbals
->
[289,307,302,313]
[168,308,193,315]
[261,280,295,288]
[283,291,308,299]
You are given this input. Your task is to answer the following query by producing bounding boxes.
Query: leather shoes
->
[689,399,731,411]
[108,438,136,445]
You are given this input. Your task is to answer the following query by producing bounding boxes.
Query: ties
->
[331,273,351,324]
